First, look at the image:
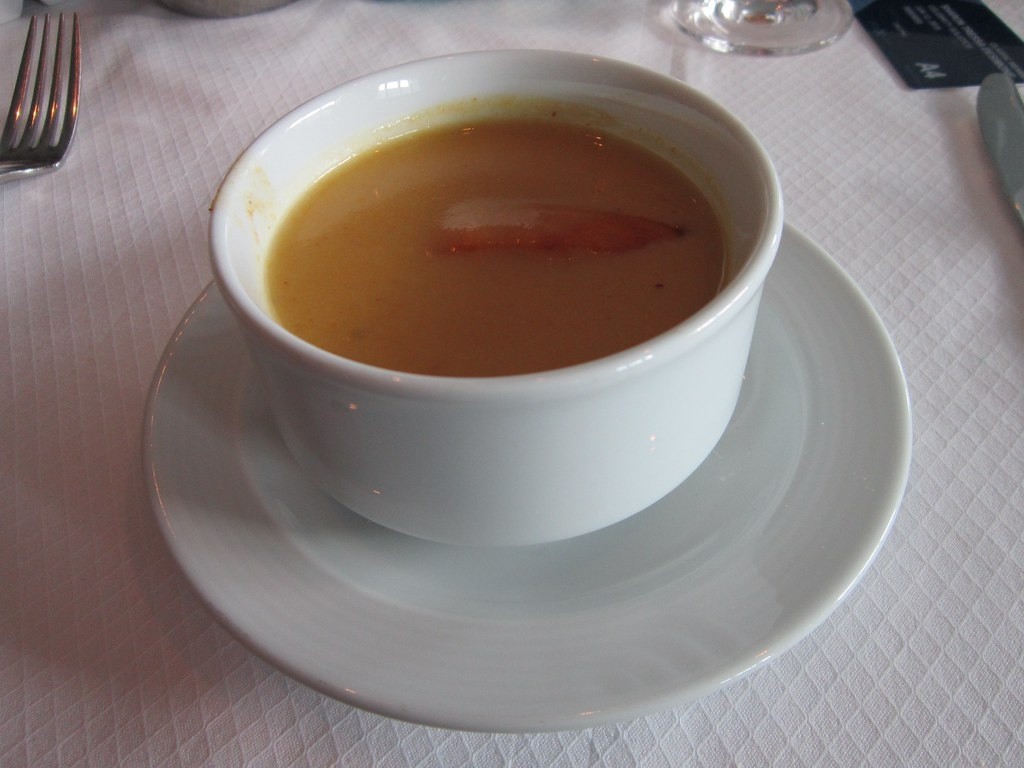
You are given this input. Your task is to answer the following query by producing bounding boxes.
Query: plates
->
[142,219,914,736]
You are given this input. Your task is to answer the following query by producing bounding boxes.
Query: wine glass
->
[672,0,853,58]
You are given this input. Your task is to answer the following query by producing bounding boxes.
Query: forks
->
[1,13,81,185]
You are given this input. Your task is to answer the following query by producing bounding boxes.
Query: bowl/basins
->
[215,54,785,546]
